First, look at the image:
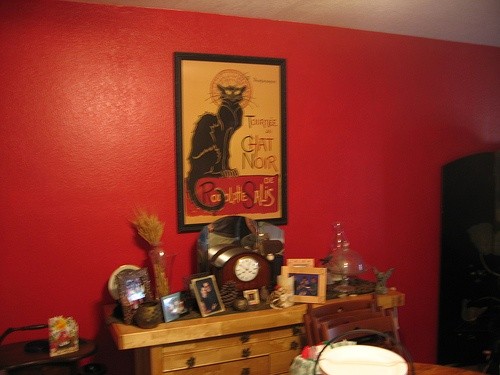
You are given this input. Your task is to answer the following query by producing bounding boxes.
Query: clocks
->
[211,246,272,291]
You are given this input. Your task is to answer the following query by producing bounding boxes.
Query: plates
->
[317,345,408,375]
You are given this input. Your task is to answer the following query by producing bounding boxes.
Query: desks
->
[407,362,489,375]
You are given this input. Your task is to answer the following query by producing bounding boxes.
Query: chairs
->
[303,293,404,356]
[0,324,106,375]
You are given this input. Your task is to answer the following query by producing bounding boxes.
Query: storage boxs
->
[47,315,78,358]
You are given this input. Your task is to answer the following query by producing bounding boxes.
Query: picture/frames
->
[173,49,289,234]
[116,267,225,325]
[243,289,260,306]
[281,258,327,305]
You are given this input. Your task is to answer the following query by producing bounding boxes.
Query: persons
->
[199,281,220,313]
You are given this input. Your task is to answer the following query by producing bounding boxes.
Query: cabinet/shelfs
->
[102,288,406,375]
[436,152,500,375]
